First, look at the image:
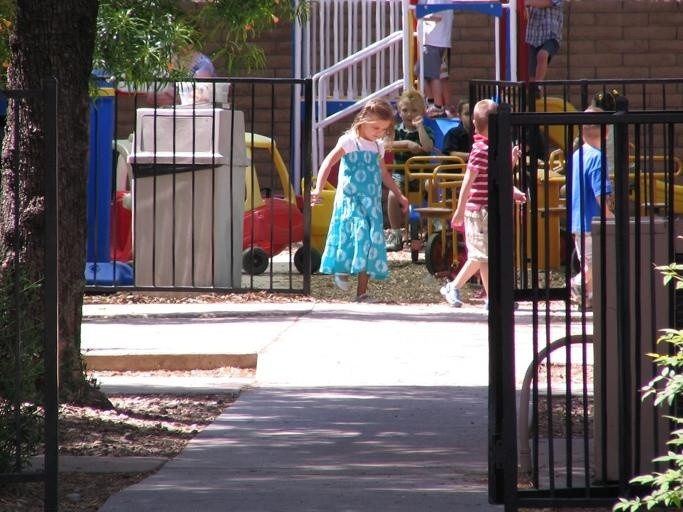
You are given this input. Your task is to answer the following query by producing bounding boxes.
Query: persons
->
[570,105,616,306]
[440,98,475,190]
[588,88,620,176]
[375,90,434,253]
[414,0,457,118]
[435,97,528,309]
[144,16,215,105]
[306,98,410,304]
[512,1,567,100]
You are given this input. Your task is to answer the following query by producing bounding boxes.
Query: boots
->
[385,231,403,250]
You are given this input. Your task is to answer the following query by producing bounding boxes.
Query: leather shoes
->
[334,273,353,291]
[357,293,380,303]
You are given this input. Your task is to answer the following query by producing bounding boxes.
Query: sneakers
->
[485,300,518,309]
[426,96,445,114]
[440,282,463,307]
